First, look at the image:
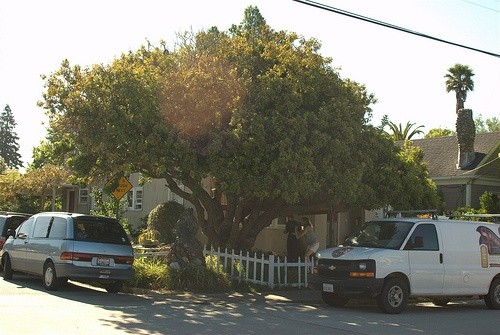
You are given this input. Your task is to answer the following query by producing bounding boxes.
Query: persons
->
[290,216,320,273]
[283,215,300,269]
[352,218,359,231]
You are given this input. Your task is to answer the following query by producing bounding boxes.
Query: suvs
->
[0,211,32,251]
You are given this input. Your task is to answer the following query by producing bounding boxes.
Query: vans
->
[1,213,137,293]
[315,220,500,314]
